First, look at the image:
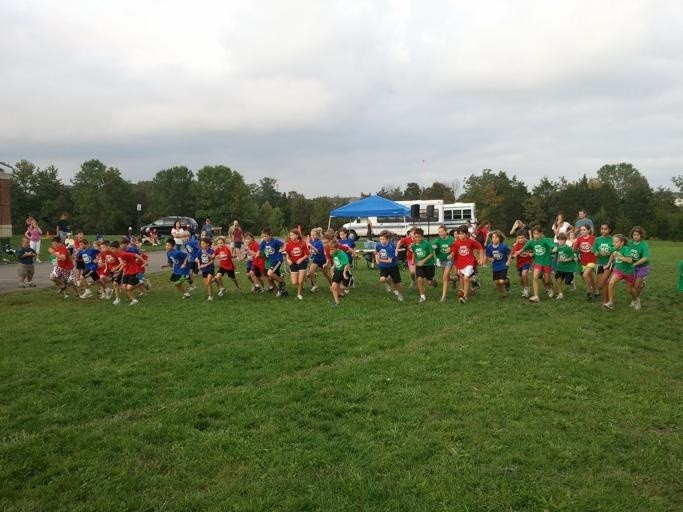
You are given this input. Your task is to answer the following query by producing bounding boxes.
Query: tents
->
[327,196,411,237]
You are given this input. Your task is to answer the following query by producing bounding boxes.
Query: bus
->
[444,201,478,239]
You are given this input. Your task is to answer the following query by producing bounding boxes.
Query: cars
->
[139,216,198,237]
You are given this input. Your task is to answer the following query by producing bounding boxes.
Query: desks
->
[352,244,439,270]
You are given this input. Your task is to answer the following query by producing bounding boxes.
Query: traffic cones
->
[44,231,50,240]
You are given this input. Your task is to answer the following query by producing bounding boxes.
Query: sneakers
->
[130,300,139,306]
[181,286,197,300]
[297,295,302,300]
[112,297,121,304]
[27,281,36,287]
[385,282,402,302]
[455,275,481,304]
[505,276,511,291]
[55,284,113,300]
[145,279,151,290]
[17,282,25,288]
[605,301,616,311]
[252,282,288,298]
[569,281,576,292]
[332,304,339,308]
[417,298,426,302]
[207,295,213,302]
[587,284,601,299]
[217,288,226,297]
[629,297,641,312]
[36,259,43,263]
[330,285,350,299]
[311,286,320,293]
[522,287,564,302]
[432,279,437,287]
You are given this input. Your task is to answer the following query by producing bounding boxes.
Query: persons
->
[159,218,245,301]
[242,226,363,306]
[507,209,651,310]
[16,213,43,288]
[367,220,490,304]
[49,211,152,305]
[143,220,161,246]
[485,230,512,298]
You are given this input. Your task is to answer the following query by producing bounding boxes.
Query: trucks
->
[341,199,444,242]
